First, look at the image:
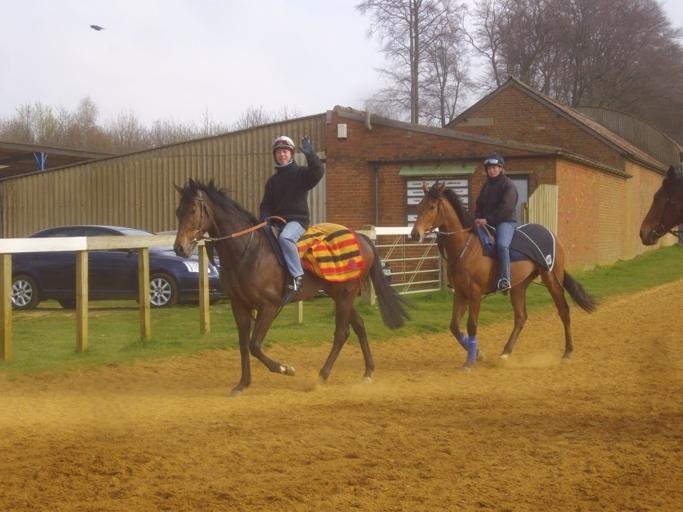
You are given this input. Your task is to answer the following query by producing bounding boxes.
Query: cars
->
[11,224,227,310]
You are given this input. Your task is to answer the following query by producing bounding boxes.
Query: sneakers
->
[288,276,304,292]
[500,282,511,297]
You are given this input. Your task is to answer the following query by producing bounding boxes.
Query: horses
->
[640,165,683,245]
[411,181,598,373]
[172,177,418,397]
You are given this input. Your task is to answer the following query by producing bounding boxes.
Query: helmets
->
[483,153,505,168]
[272,136,295,152]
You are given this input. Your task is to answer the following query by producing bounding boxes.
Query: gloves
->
[296,135,316,155]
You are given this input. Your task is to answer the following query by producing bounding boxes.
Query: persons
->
[258,133,325,305]
[446,152,519,292]
